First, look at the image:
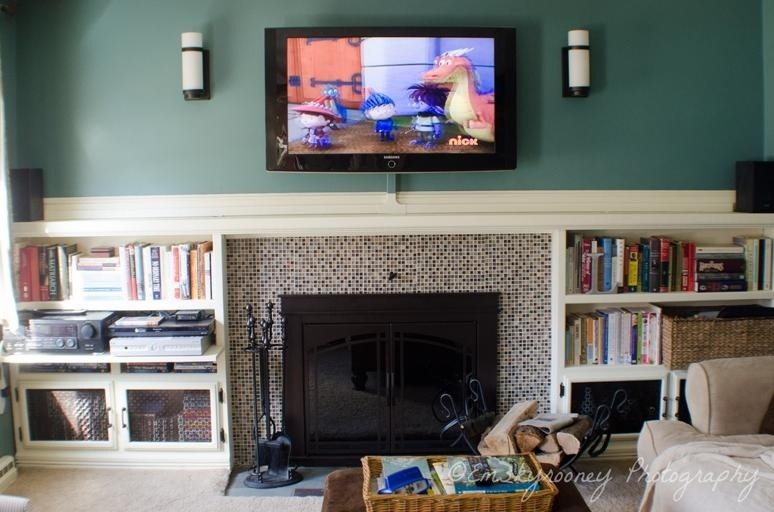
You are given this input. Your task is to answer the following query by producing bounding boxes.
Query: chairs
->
[636,353,774,512]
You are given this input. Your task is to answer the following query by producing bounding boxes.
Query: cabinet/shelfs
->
[0,232,230,471]
[557,222,774,462]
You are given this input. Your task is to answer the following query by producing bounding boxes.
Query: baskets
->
[659,303,774,371]
[358,452,560,512]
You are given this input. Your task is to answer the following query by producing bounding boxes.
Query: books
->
[114,315,164,326]
[376,452,543,498]
[566,231,772,368]
[11,239,211,305]
[20,362,211,441]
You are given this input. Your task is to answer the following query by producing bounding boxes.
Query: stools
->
[318,461,591,512]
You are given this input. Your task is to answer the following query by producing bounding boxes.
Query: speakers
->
[735,160,774,213]
[10,167,42,220]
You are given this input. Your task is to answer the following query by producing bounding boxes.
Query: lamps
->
[180,31,211,102]
[560,28,593,99]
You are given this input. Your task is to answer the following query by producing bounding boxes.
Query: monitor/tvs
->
[262,26,517,173]
